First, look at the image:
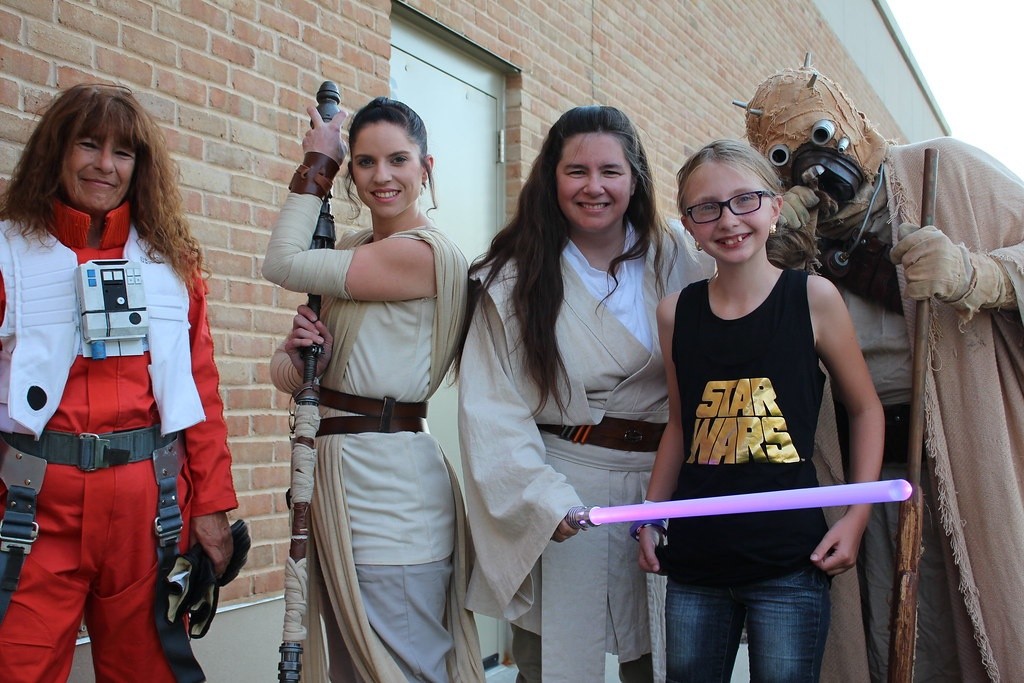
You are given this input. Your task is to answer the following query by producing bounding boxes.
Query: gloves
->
[887,219,972,305]
[775,183,823,242]
[164,520,251,638]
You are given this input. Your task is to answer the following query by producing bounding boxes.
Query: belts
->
[315,385,431,437]
[535,416,667,453]
[835,404,927,465]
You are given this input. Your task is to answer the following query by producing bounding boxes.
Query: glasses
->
[685,190,776,225]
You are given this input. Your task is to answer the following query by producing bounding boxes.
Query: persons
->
[0,84,239,683]
[458,54,1024,683]
[261,96,486,683]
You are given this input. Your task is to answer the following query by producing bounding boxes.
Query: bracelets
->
[630,500,668,544]
